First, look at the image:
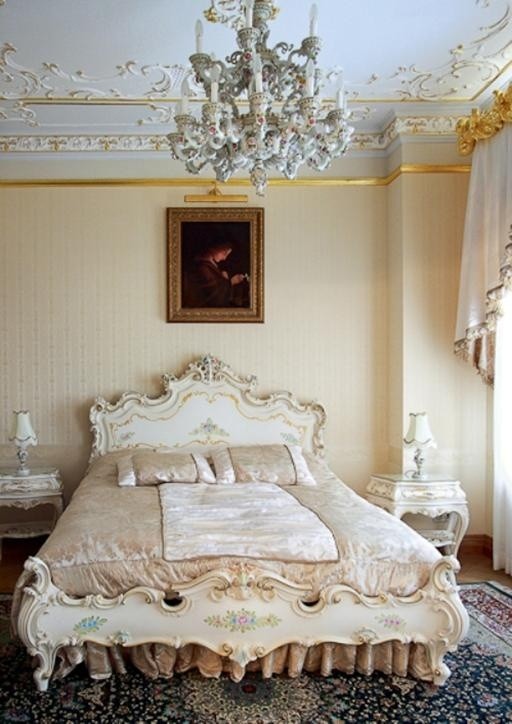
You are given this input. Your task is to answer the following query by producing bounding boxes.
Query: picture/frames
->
[164,206,266,324]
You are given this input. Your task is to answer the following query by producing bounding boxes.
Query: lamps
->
[7,408,39,472]
[165,1,359,196]
[402,410,437,481]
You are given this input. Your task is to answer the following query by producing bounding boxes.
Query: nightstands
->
[0,465,65,540]
[364,473,472,559]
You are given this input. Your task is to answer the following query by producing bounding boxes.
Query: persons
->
[182,241,247,307]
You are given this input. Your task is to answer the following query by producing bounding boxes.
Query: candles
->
[208,65,221,103]
[173,103,179,117]
[344,96,349,113]
[179,78,191,117]
[251,56,264,93]
[309,3,320,37]
[335,74,344,109]
[244,1,255,28]
[306,58,317,99]
[194,18,205,54]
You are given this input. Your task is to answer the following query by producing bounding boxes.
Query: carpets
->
[1,578,512,724]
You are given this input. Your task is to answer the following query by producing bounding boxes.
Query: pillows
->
[115,449,216,488]
[210,444,317,486]
[9,354,471,692]
